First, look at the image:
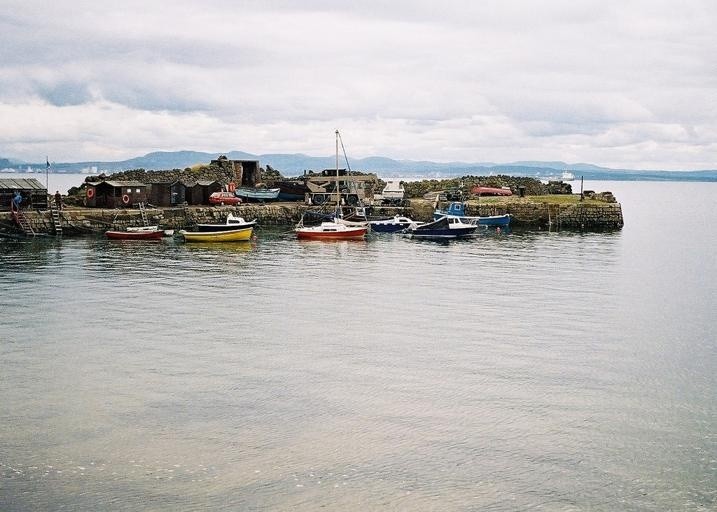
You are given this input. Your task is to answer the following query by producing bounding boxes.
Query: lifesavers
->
[87,189,93,197]
[123,195,129,203]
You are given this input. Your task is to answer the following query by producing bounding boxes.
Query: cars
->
[209,192,242,206]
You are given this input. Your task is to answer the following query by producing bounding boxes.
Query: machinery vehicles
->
[305,174,379,206]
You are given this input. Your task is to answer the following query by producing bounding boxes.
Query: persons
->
[54,191,62,211]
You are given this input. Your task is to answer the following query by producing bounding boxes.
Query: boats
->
[104,213,257,243]
[383,181,405,199]
[234,188,280,199]
[561,167,576,181]
[369,203,514,241]
[293,129,371,240]
